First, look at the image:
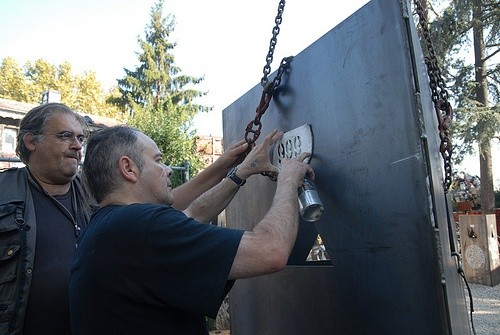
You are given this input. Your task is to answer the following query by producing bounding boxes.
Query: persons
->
[70,124,317,335]
[0,101,261,333]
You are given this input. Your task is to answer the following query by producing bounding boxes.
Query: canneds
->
[296,174,325,222]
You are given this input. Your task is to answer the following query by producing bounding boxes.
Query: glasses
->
[42,132,85,145]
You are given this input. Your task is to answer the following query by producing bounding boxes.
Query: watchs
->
[226,166,246,186]
